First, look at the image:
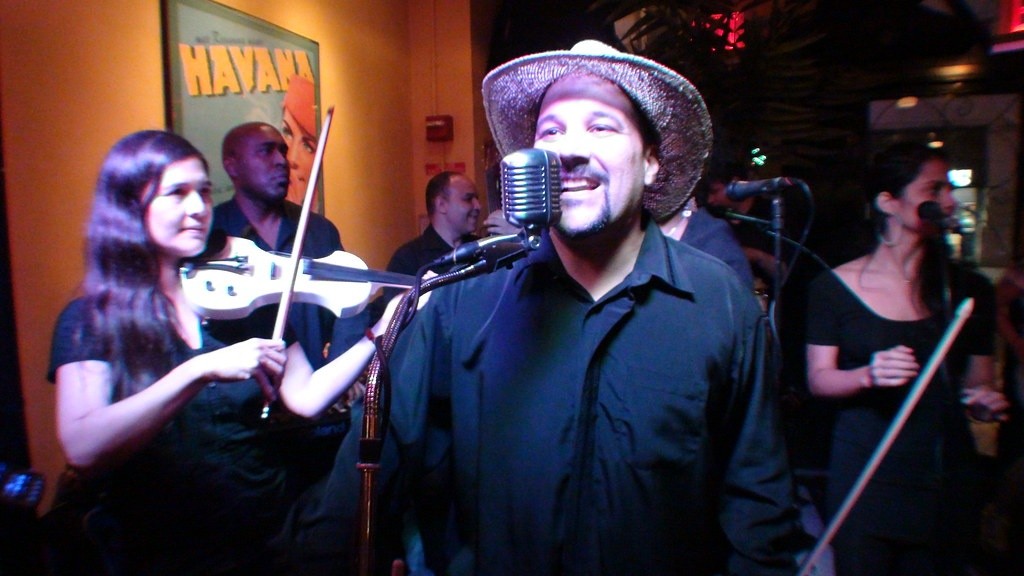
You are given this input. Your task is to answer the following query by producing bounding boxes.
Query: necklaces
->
[885,244,913,284]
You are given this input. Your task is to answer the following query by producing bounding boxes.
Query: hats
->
[482,39,712,222]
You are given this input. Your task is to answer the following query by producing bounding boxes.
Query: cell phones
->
[4,473,44,509]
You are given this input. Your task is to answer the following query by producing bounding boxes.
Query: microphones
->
[723,175,799,203]
[434,148,564,270]
[917,200,964,231]
[710,205,772,226]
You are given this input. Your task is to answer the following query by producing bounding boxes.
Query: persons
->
[1,123,438,576]
[275,73,321,215]
[260,39,804,576]
[804,149,1024,576]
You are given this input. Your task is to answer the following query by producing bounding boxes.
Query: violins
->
[178,235,429,320]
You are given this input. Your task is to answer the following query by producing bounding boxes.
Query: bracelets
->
[364,328,377,346]
